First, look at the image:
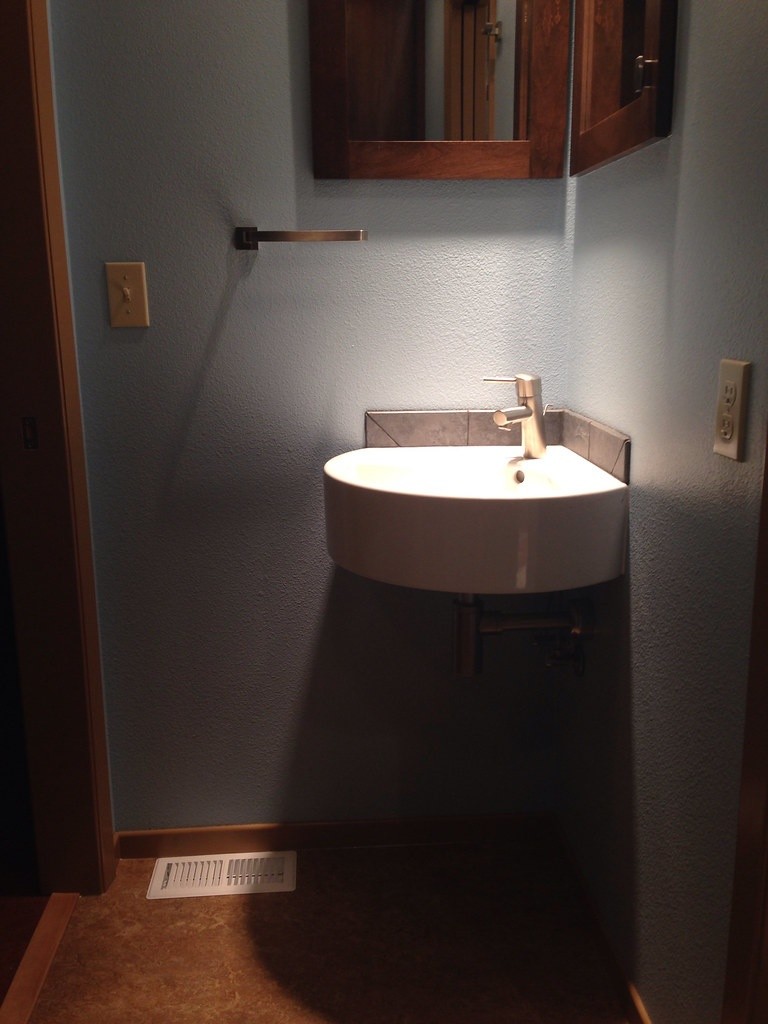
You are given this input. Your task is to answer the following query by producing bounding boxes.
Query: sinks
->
[322,443,629,597]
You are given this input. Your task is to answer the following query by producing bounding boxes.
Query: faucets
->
[482,372,550,460]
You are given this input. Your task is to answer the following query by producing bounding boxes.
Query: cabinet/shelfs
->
[308,0,682,182]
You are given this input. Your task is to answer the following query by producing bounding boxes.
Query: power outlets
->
[713,359,748,459]
